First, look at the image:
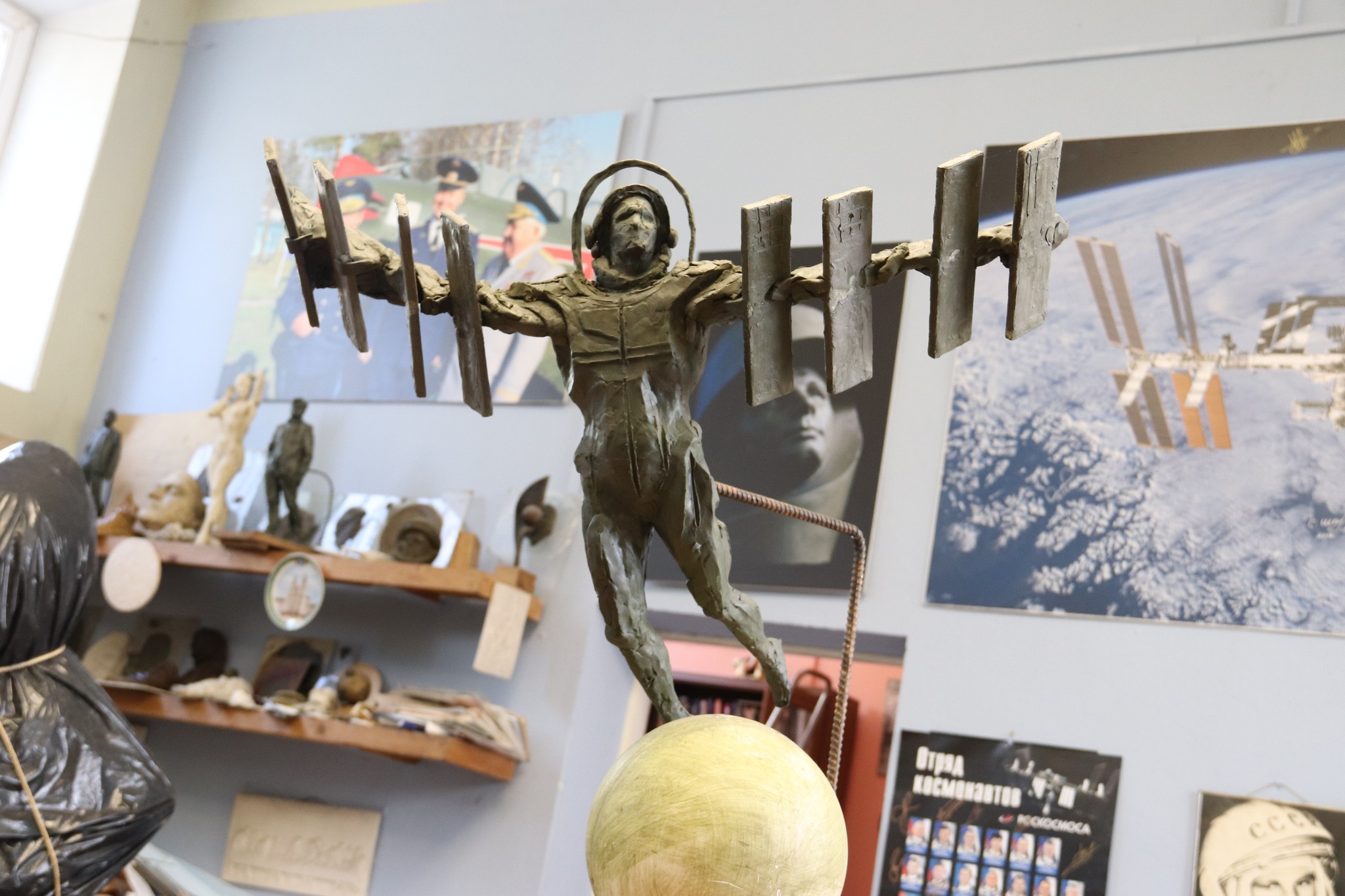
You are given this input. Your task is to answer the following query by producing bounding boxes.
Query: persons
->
[285,185,1068,736]
[263,397,314,550]
[194,370,267,548]
[260,153,567,407]
[80,410,122,516]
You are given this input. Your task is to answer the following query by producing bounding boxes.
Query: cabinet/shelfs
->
[77,533,544,777]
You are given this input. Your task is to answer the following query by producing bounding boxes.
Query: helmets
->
[675,301,832,418]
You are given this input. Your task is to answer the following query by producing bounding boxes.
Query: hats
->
[337,175,373,213]
[438,156,478,191]
[507,183,560,228]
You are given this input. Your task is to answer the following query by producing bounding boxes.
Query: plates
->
[261,551,325,632]
